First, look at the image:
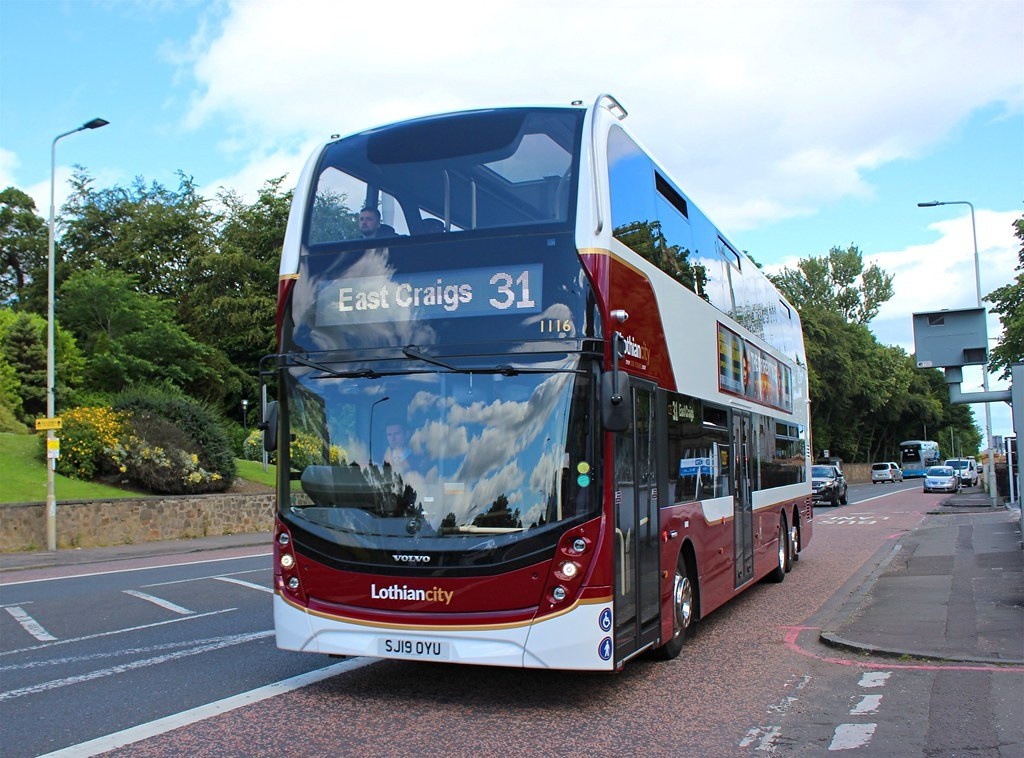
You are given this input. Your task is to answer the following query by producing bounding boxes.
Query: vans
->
[943,455,978,488]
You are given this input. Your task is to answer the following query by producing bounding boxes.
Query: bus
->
[259,91,818,679]
[899,439,941,479]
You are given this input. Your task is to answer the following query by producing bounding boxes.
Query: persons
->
[743,355,748,392]
[374,420,432,477]
[359,207,400,240]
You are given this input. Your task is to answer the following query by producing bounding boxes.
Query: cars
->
[977,462,983,473]
[923,465,959,494]
[811,464,850,508]
[870,461,904,485]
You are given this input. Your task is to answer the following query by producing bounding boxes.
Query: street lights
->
[43,117,109,554]
[916,199,1000,510]
